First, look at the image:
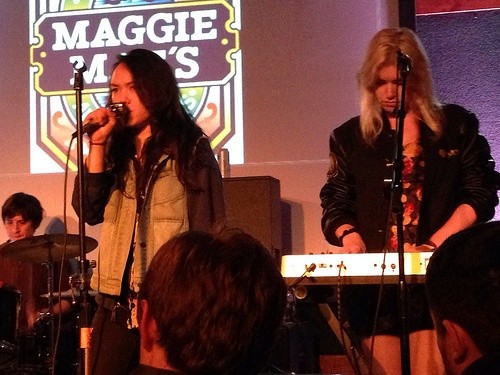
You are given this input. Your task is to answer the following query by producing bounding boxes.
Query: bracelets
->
[88,141,107,145]
[337,227,358,242]
[423,239,438,250]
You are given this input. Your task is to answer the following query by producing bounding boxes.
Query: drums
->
[0,282,24,366]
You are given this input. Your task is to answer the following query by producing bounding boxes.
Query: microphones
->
[72,102,129,138]
[395,48,414,71]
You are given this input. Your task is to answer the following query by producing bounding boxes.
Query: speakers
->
[222,176,282,257]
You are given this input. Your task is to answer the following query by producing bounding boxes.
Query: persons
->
[423,220,500,375]
[318,29,500,375]
[0,192,82,320]
[70,46,226,375]
[133,226,290,375]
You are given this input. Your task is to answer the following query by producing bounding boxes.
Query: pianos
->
[279,250,434,285]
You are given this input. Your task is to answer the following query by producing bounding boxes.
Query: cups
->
[218,149,229,177]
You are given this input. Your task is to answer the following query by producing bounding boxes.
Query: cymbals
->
[0,234,99,264]
[40,290,99,299]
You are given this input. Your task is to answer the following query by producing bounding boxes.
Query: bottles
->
[285,279,296,324]
